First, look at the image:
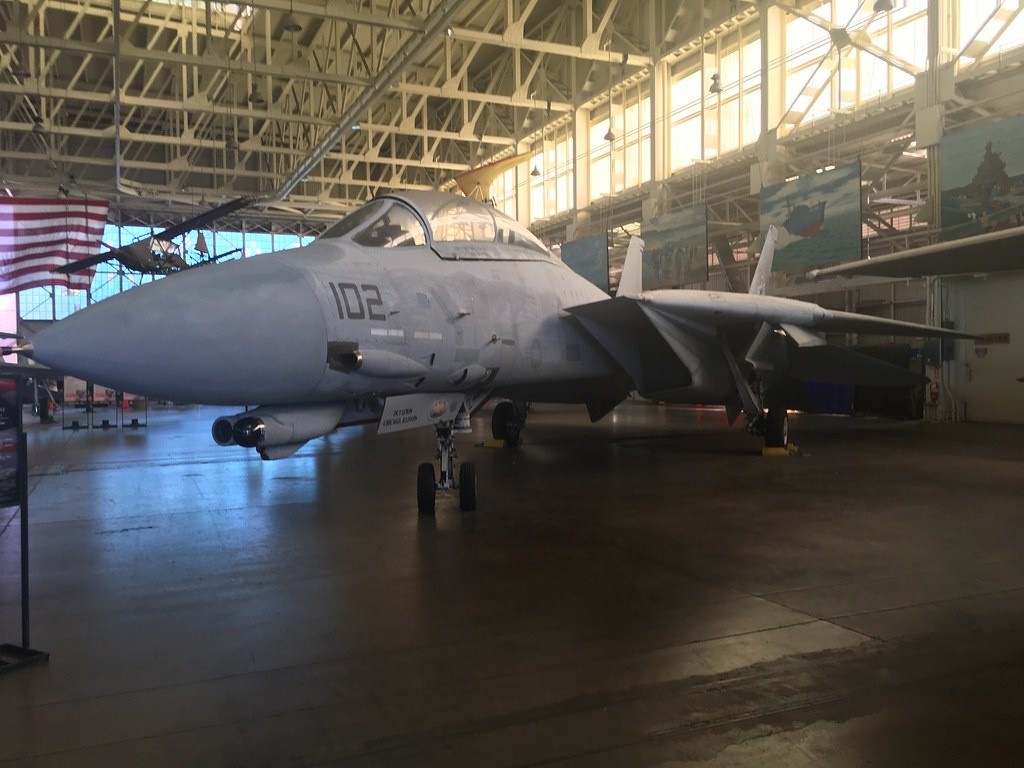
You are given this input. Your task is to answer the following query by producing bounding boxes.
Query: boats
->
[780,201,827,237]
[964,140,1011,199]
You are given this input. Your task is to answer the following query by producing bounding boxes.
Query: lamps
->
[33,0,46,135]
[44,158,56,169]
[283,0,305,32]
[225,0,237,149]
[709,74,723,92]
[531,164,541,176]
[872,0,894,11]
[199,194,207,206]
[604,128,616,141]
[249,0,266,103]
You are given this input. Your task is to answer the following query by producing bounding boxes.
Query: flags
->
[1,195,109,295]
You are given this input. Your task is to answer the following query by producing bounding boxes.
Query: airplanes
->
[47,197,252,274]
[0,191,984,512]
